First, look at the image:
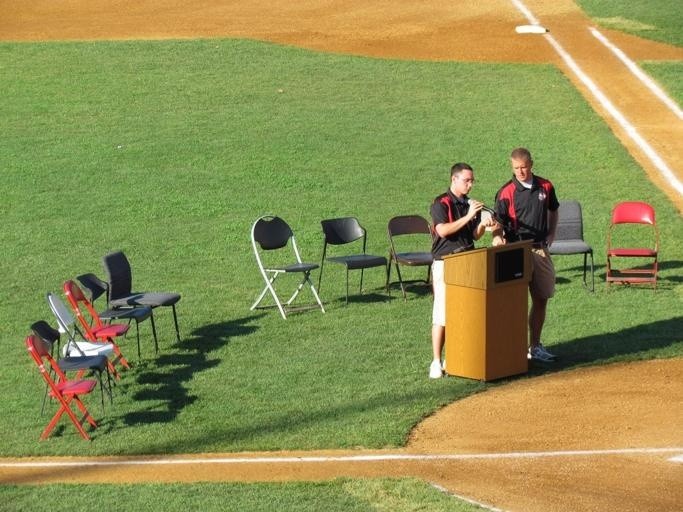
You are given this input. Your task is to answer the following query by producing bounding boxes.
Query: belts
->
[532,242,547,250]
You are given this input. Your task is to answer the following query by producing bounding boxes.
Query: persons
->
[491,147,561,363]
[426,162,496,378]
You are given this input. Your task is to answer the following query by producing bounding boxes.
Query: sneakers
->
[429,358,443,379]
[528,342,560,363]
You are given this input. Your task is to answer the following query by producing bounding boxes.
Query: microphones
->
[468,198,495,219]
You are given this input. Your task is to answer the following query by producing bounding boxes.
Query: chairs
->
[29,253,181,442]
[249,214,325,319]
[606,202,659,292]
[547,199,595,291]
[386,215,437,302]
[317,218,392,306]
